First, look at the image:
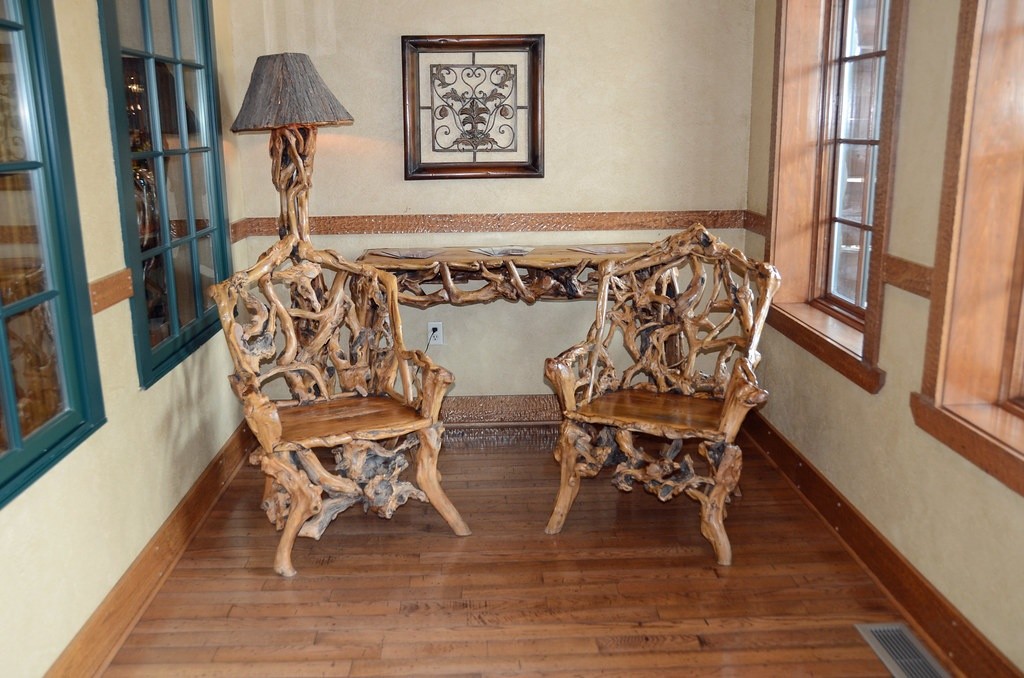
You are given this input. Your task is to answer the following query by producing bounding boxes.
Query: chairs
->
[541,223,782,566]
[207,231,473,576]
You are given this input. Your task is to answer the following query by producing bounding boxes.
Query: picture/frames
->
[401,33,546,181]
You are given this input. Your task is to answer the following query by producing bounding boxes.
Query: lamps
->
[121,55,200,347]
[230,52,358,229]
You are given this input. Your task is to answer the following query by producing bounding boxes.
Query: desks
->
[350,240,684,373]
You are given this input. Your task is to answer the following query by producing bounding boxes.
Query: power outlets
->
[427,322,442,345]
